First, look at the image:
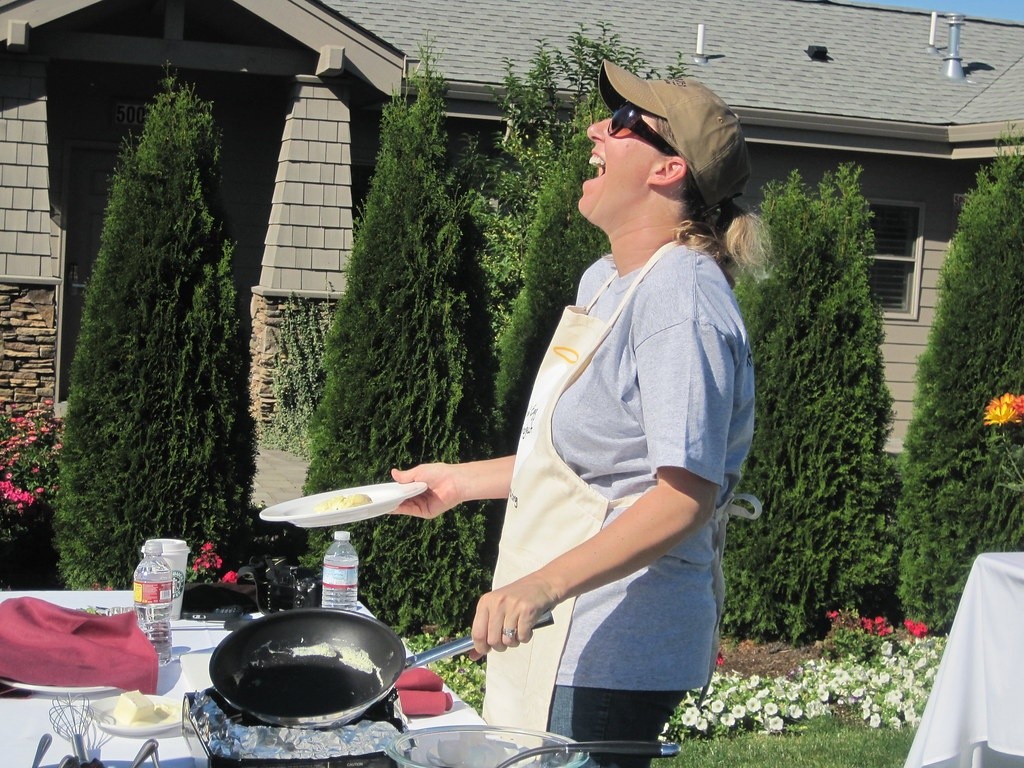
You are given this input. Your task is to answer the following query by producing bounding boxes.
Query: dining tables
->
[0,591,488,768]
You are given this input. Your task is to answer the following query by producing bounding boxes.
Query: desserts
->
[112,690,155,727]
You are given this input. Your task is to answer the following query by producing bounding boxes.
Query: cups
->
[142,539,191,620]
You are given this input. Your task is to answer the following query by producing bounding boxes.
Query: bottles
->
[133,540,173,666]
[322,531,359,611]
[77,606,133,616]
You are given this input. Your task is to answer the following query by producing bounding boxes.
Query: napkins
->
[0,596,158,695]
[395,668,454,715]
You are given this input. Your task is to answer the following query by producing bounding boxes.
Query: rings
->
[502,628,515,639]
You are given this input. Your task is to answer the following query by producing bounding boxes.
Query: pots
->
[209,608,554,728]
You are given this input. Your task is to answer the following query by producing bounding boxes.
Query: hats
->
[598,59,751,208]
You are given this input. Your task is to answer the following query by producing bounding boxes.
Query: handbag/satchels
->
[180,551,322,620]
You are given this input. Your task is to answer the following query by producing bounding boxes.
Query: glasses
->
[608,102,680,157]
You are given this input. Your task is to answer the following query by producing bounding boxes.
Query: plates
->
[0,677,117,694]
[259,482,428,528]
[85,694,182,736]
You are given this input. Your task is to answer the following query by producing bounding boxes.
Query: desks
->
[904,552,1024,768]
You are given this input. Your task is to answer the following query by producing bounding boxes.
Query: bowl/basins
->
[385,725,590,768]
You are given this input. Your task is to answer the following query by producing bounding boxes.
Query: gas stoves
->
[181,688,414,768]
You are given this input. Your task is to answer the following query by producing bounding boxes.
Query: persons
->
[379,81,758,768]
